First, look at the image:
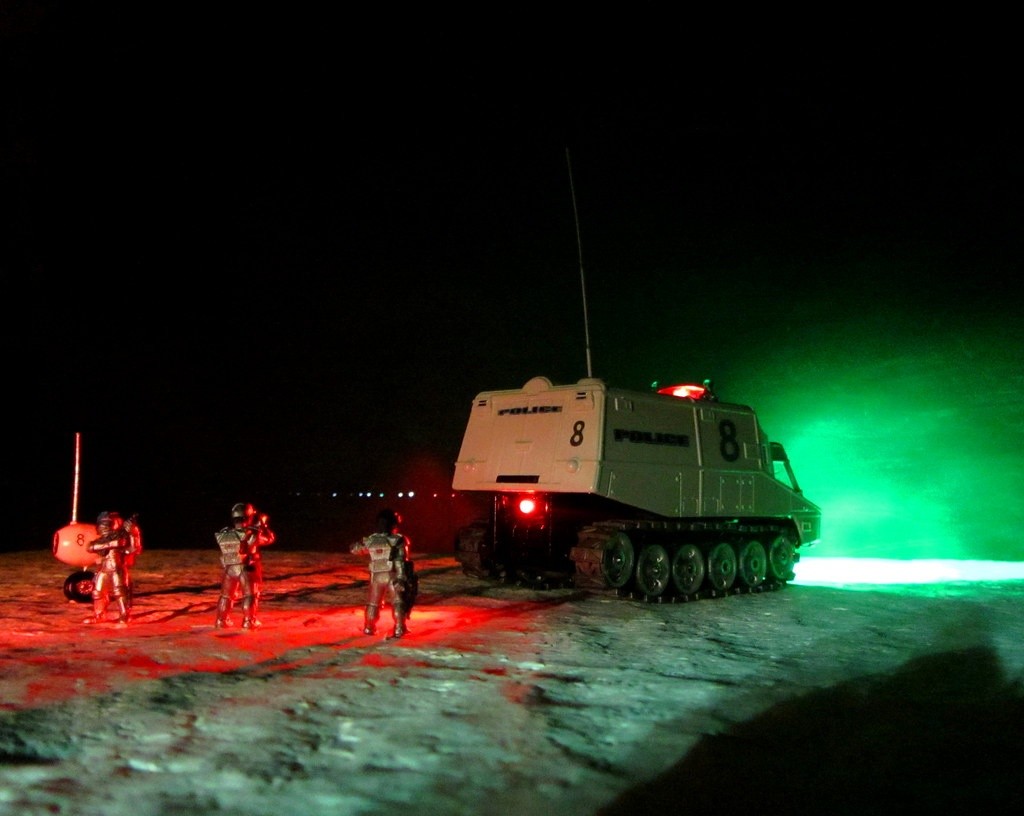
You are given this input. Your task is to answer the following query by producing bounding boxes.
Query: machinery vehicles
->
[449,146,822,601]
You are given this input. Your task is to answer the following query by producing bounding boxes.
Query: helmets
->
[232,504,257,517]
[97,511,121,535]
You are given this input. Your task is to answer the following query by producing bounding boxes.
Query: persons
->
[216,501,274,631]
[83,510,137,626]
[352,510,411,638]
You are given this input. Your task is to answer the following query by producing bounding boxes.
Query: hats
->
[377,509,402,524]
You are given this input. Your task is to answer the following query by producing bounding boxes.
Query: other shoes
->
[83,613,105,624]
[216,618,235,628]
[395,628,403,637]
[116,614,131,624]
[366,626,378,635]
[244,619,262,628]
